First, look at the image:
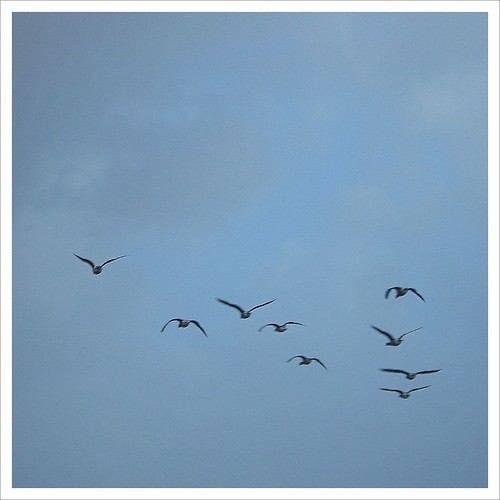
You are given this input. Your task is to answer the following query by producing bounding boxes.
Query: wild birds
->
[160,318,208,338]
[258,321,309,334]
[378,366,444,380]
[370,324,424,347]
[286,354,328,371]
[384,285,426,303]
[378,384,432,400]
[71,252,131,276]
[215,296,279,320]
